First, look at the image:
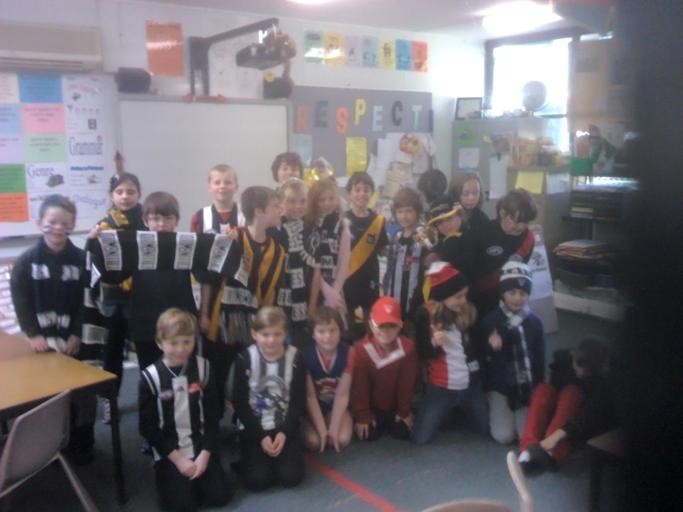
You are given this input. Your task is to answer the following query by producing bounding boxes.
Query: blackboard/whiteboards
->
[116,93,293,241]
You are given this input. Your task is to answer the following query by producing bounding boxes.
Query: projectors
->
[235,43,295,69]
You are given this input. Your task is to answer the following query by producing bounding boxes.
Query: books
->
[569,188,625,222]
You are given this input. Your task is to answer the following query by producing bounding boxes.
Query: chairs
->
[505,450,535,511]
[0,388,103,512]
[424,500,514,511]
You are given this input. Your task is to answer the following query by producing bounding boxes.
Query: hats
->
[498,261,533,294]
[371,297,404,330]
[425,266,467,298]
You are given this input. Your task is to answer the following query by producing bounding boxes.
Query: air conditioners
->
[0,18,102,75]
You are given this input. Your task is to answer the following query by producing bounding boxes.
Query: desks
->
[0,329,116,509]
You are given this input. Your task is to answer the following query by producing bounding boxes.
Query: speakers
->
[264,77,293,99]
[115,68,151,91]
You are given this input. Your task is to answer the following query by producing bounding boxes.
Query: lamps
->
[187,17,294,92]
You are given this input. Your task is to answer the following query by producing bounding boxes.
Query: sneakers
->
[97,395,121,425]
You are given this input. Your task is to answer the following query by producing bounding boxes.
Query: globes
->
[520,81,550,119]
